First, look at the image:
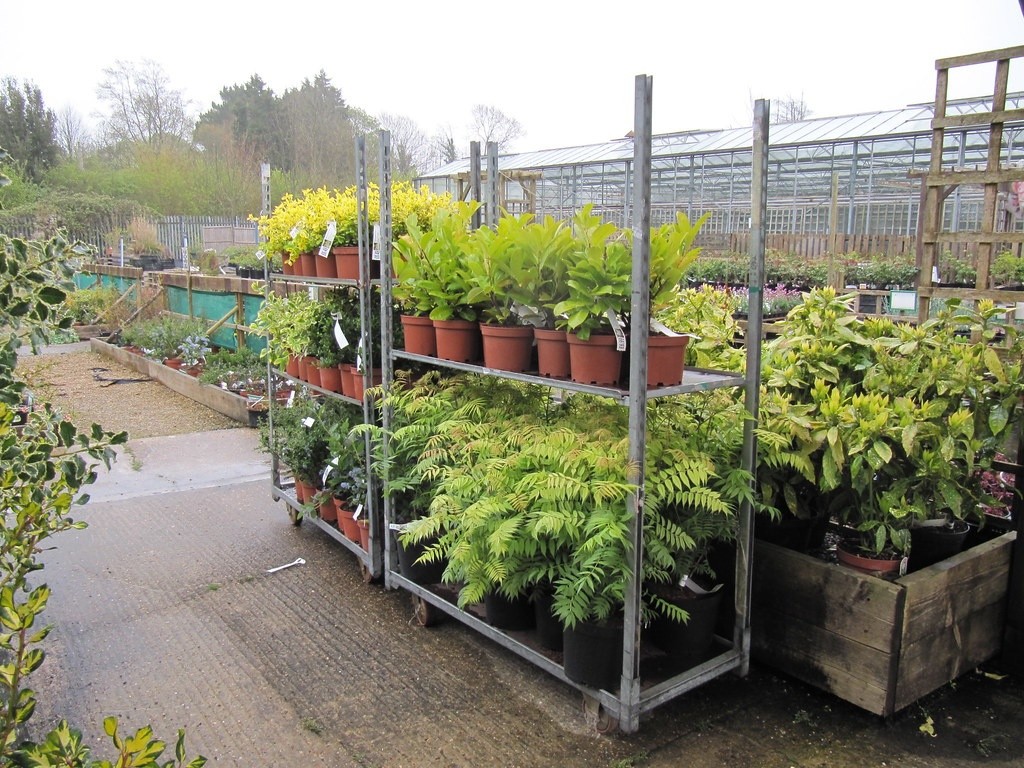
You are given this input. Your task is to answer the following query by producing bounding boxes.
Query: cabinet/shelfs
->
[377,74,769,735]
[260,129,481,582]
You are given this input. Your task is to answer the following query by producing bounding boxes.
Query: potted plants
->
[242,280,426,402]
[118,312,294,402]
[389,207,711,388]
[667,280,1024,578]
[256,389,387,554]
[346,368,811,692]
[221,182,455,280]
